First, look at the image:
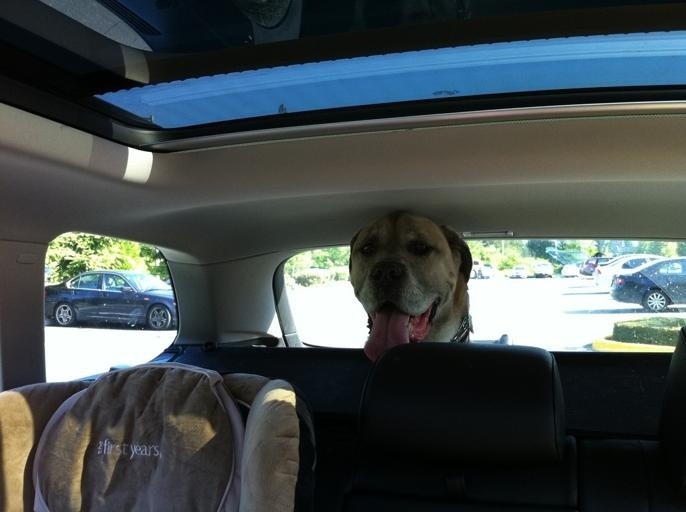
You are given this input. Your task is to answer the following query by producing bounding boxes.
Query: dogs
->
[348,210,473,367]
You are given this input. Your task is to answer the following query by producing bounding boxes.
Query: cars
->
[468,254,633,282]
[43,270,178,333]
[607,257,685,314]
[593,254,667,286]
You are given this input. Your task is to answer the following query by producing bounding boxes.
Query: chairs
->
[348,343,582,510]
[160,346,316,512]
[632,329,684,510]
